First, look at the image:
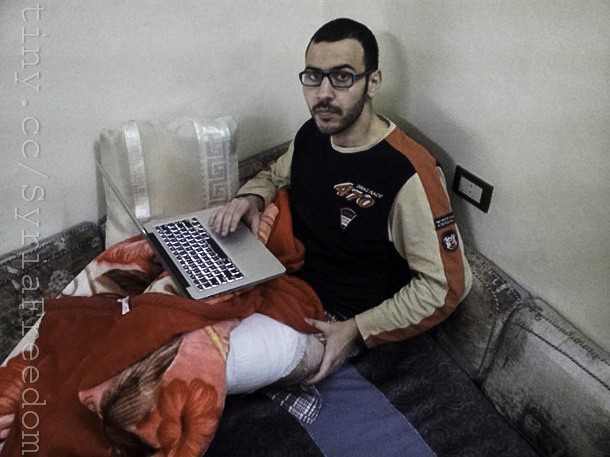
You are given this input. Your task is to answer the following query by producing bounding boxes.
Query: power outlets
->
[454,165,498,214]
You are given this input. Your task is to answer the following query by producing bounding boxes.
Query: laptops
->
[94,160,288,302]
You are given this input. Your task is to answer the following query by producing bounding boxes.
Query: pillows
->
[99,119,243,248]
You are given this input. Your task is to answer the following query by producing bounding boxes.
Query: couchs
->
[0,140,595,450]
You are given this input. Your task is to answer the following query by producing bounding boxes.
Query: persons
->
[207,18,473,385]
